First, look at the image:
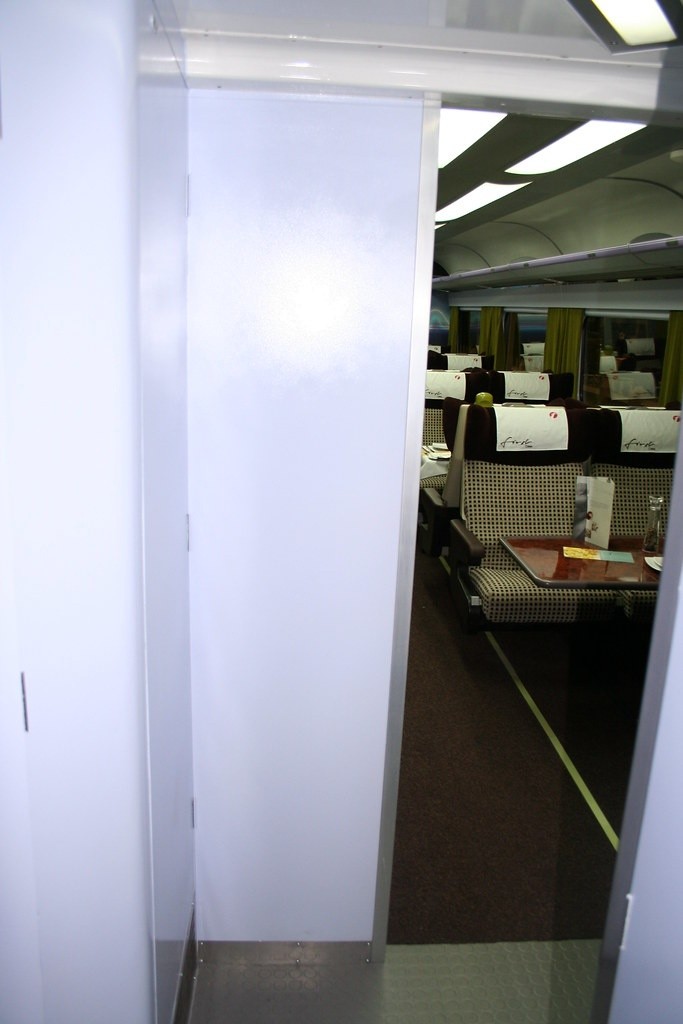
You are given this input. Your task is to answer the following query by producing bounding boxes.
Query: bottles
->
[642,495,664,553]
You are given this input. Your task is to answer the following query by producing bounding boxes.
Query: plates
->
[429,453,450,459]
[644,556,663,571]
[433,443,447,449]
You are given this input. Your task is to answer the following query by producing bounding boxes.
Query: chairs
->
[586,407,683,629]
[518,336,664,401]
[448,403,625,628]
[419,343,666,557]
